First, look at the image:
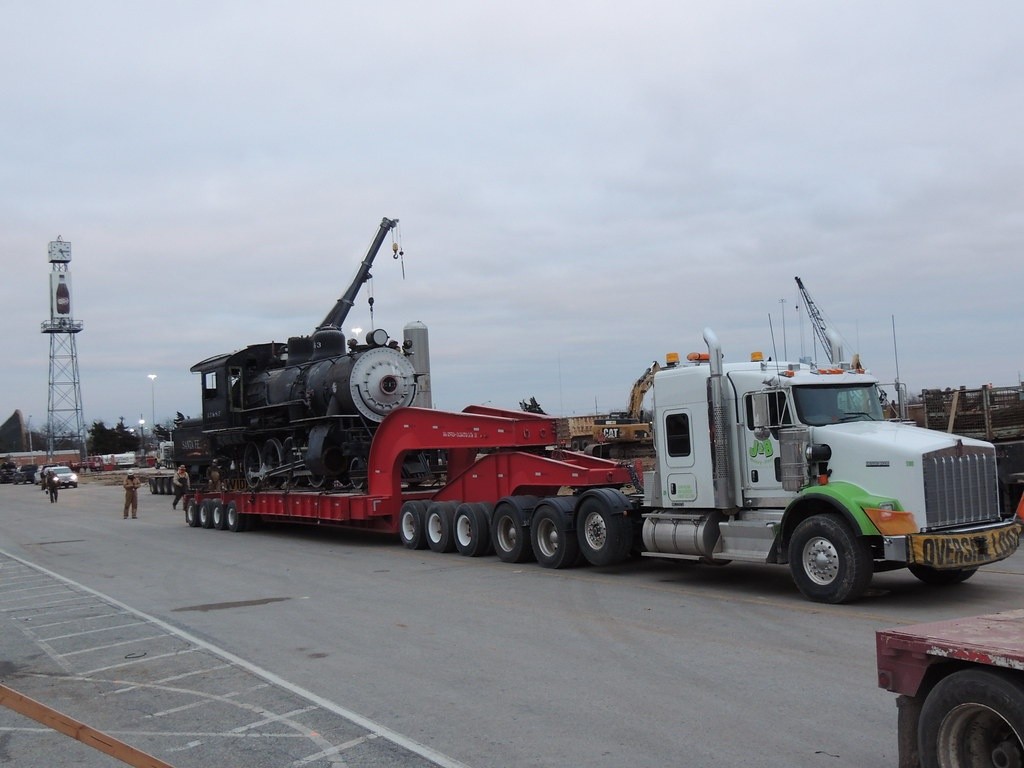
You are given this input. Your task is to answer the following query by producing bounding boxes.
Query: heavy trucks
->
[173,325,1022,606]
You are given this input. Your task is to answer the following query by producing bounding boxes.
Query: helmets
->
[127,470,134,475]
[50,468,54,471]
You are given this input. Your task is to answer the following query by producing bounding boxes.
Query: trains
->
[171,215,429,492]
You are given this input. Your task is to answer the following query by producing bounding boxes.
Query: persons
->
[122,469,141,519]
[48,473,59,503]
[172,465,191,510]
[40,470,47,491]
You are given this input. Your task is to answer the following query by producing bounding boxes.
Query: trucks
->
[71,456,103,472]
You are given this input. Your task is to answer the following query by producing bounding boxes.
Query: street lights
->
[146,374,157,430]
[139,419,145,448]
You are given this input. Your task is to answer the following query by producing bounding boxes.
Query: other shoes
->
[132,516,137,519]
[124,516,128,519]
[173,504,176,509]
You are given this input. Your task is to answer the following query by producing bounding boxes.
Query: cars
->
[0,452,79,489]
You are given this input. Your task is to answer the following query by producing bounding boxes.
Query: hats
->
[178,465,186,469]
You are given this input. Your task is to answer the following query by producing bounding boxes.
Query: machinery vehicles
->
[584,360,663,460]
